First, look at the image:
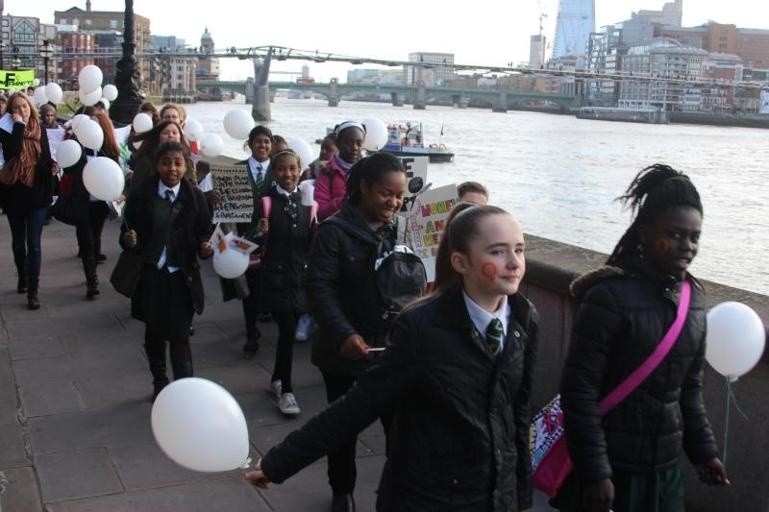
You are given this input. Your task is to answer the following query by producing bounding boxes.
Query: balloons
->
[32,65,155,202]
[704,302,766,382]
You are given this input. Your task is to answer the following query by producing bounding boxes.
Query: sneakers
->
[330,493,356,512]
[151,378,171,402]
[270,376,282,399]
[277,393,301,414]
[16,274,27,293]
[26,291,41,311]
[294,313,312,342]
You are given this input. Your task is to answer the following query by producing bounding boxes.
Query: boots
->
[77,248,109,299]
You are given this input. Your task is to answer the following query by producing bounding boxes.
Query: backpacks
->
[317,211,431,364]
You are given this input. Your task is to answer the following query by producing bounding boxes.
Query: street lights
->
[11,46,20,70]
[37,39,53,86]
[0,40,6,69]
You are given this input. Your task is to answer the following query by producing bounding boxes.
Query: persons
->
[457,182,489,205]
[246,203,540,512]
[549,163,731,512]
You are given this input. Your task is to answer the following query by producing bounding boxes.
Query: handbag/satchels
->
[107,249,142,298]
[527,393,579,500]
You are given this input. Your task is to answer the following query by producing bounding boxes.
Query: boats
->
[313,118,453,165]
[575,106,670,125]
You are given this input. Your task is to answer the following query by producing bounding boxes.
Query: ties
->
[164,190,178,284]
[255,164,265,196]
[484,318,504,359]
